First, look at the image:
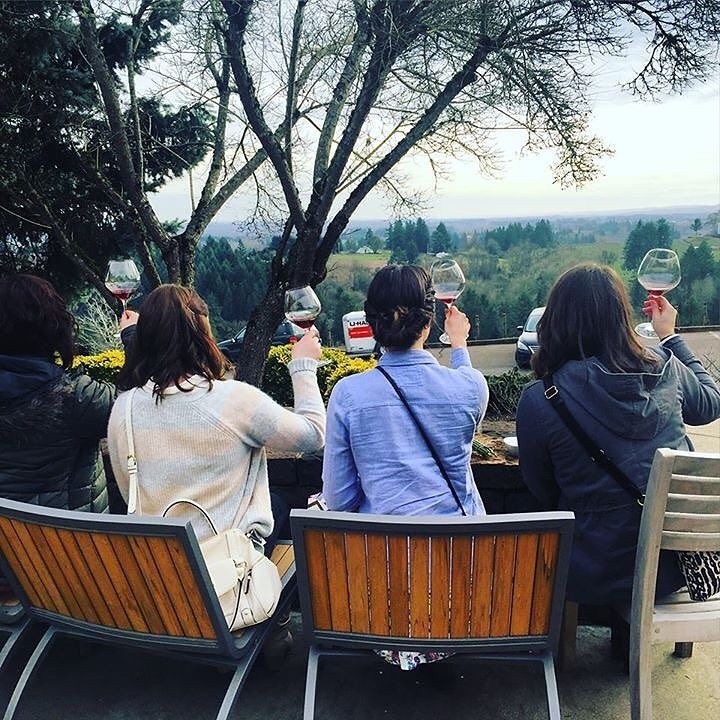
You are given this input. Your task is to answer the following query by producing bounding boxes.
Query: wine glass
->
[284,285,332,367]
[633,249,681,339]
[428,259,469,345]
[103,259,141,338]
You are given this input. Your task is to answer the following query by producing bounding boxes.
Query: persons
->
[516,265,720,604]
[322,265,490,671]
[108,282,327,561]
[0,275,141,516]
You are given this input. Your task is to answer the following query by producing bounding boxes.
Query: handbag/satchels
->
[161,498,282,634]
[677,550,720,602]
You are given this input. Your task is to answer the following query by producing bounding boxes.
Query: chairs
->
[289,509,575,720]
[565,448,720,720]
[0,498,297,720]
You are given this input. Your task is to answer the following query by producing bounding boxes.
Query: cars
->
[215,321,305,364]
[514,308,547,370]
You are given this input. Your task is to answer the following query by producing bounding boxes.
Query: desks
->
[100,413,526,539]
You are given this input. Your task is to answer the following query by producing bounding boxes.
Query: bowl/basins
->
[503,436,518,456]
